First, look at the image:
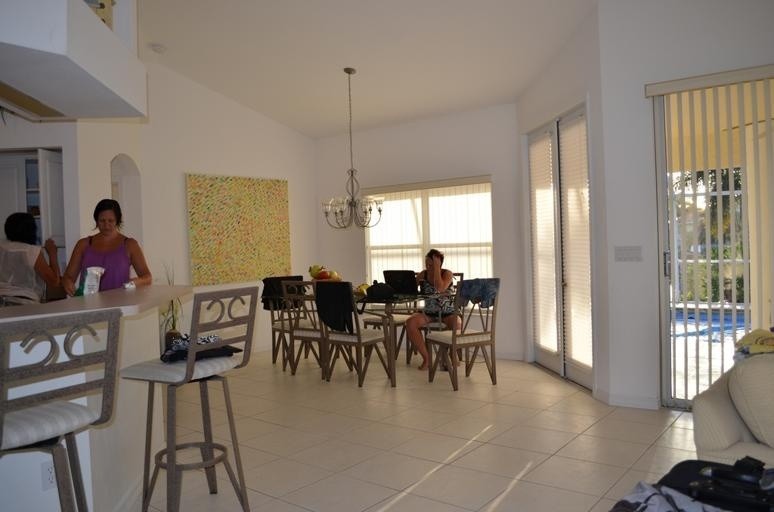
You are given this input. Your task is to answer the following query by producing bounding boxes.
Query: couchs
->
[693,332,773,469]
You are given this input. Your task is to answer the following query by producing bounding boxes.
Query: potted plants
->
[157,258,186,350]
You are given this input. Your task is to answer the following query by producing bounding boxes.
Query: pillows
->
[728,351,774,447]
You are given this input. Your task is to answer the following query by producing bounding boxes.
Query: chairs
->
[0,306,122,512]
[427,277,502,392]
[118,283,259,512]
[264,276,308,360]
[282,280,323,378]
[364,270,412,358]
[408,273,464,359]
[317,278,387,386]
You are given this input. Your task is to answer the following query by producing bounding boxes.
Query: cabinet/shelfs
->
[0,148,65,247]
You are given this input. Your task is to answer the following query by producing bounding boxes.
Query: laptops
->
[383,270,419,295]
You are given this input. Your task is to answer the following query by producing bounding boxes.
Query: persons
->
[406,249,461,369]
[61,200,152,296]
[0,212,61,307]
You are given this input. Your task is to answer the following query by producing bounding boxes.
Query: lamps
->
[321,68,382,230]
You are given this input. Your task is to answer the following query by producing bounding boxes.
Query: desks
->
[610,459,774,511]
[289,290,456,386]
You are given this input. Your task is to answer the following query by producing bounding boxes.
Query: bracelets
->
[123,280,136,291]
